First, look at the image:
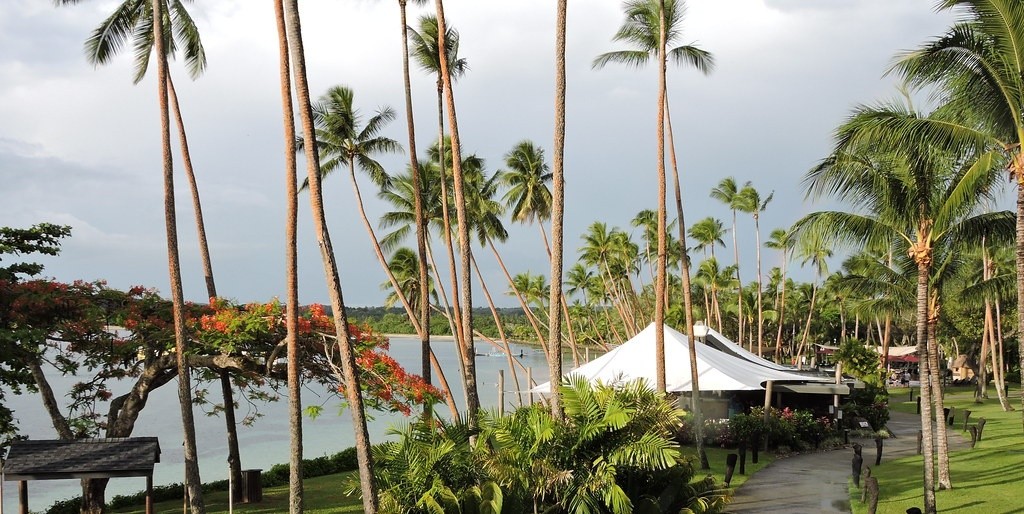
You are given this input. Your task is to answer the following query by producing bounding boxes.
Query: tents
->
[529,321,839,393]
